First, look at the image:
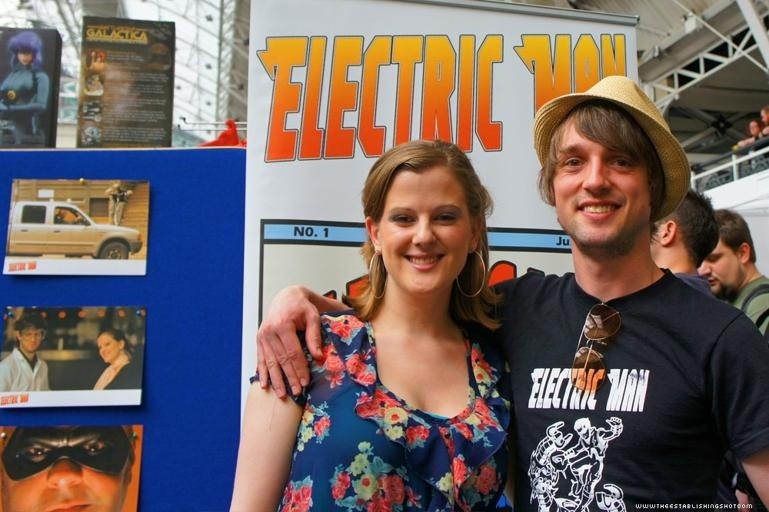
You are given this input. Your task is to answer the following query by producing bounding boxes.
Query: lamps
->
[679,9,706,35]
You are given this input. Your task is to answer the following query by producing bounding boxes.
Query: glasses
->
[571,302,622,391]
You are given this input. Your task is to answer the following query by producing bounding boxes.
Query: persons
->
[219,136,521,510]
[0,423,141,511]
[0,28,51,147]
[0,305,55,394]
[83,329,142,390]
[695,209,769,350]
[759,105,769,138]
[648,186,720,297]
[735,117,769,174]
[249,73,769,512]
[55,209,82,225]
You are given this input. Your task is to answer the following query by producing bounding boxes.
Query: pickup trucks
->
[6,199,142,259]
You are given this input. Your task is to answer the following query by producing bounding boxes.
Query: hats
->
[534,76,690,220]
[7,31,43,70]
